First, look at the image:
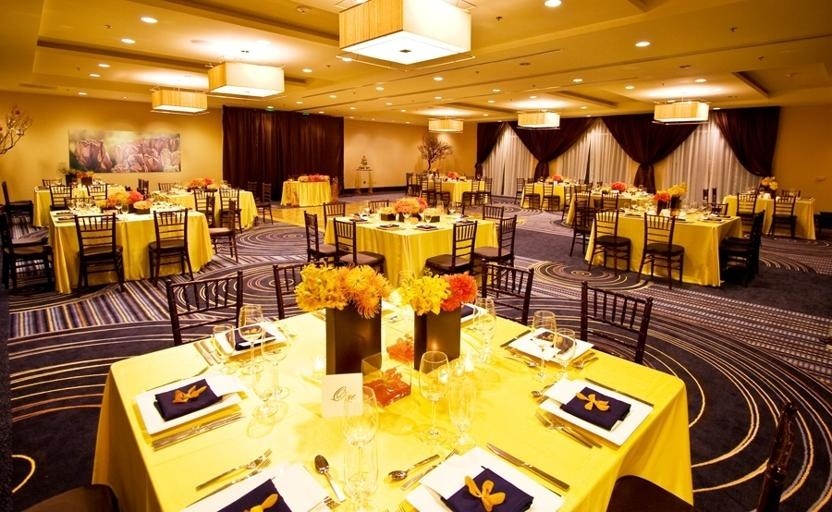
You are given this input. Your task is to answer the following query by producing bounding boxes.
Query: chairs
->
[0,178,274,299]
[19,257,795,512]
[282,164,820,289]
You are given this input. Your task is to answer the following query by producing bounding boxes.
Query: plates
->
[402,447,563,512]
[542,384,654,448]
[457,304,482,323]
[181,460,332,512]
[508,326,596,365]
[136,384,240,435]
[351,215,475,231]
[622,207,734,223]
[52,209,122,224]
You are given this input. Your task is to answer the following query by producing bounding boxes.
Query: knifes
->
[585,378,656,408]
[150,409,247,452]
[486,441,570,491]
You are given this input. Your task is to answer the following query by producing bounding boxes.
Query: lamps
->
[428,114,464,134]
[150,80,211,117]
[652,97,709,128]
[516,106,561,130]
[336,0,473,70]
[206,50,285,99]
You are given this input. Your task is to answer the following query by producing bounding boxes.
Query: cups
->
[65,196,130,217]
[362,202,462,224]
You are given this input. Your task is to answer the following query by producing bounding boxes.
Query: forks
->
[186,458,270,507]
[536,411,605,449]
[194,448,272,491]
[400,447,459,491]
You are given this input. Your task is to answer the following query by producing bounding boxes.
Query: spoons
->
[313,452,346,503]
[389,452,439,482]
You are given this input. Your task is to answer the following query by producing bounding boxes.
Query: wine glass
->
[472,296,498,362]
[531,310,576,382]
[209,301,291,421]
[414,350,478,452]
[338,385,381,509]
